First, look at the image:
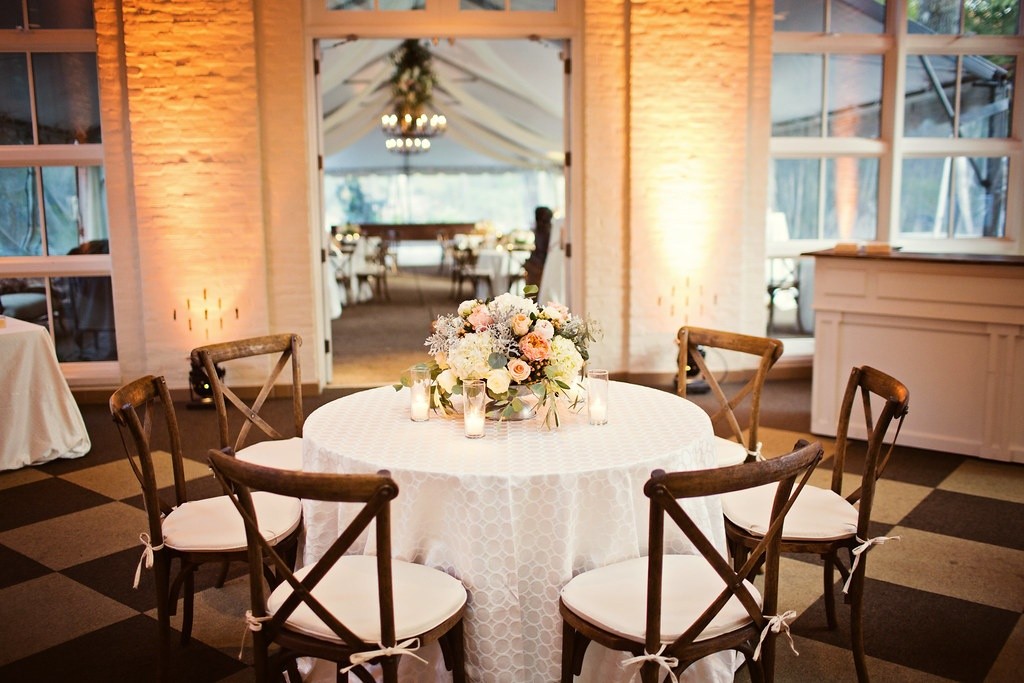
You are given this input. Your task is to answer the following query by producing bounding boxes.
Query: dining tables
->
[473,247,531,302]
[303,374,736,683]
[65,238,117,359]
[0,314,91,471]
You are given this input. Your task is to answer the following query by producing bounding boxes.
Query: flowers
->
[387,70,432,111]
[392,285,604,432]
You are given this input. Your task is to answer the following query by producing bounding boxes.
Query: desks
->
[362,223,475,239]
[801,247,1024,463]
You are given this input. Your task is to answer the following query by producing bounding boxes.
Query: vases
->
[485,379,546,421]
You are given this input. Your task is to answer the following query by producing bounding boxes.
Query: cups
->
[587,369,609,426]
[462,380,486,438]
[410,367,432,422]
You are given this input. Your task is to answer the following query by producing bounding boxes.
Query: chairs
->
[190,333,303,589]
[0,286,66,339]
[557,439,824,683]
[719,364,910,682]
[764,210,802,322]
[328,220,534,320]
[677,326,784,575]
[206,447,468,683]
[107,374,302,646]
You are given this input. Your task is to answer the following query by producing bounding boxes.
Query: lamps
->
[186,363,228,410]
[380,38,448,155]
[674,341,710,395]
